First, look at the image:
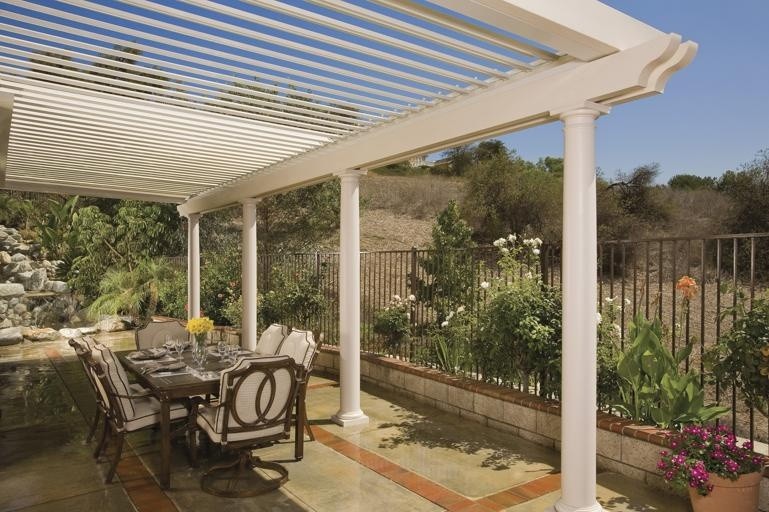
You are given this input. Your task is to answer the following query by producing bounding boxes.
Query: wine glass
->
[165,335,208,373]
[217,340,238,368]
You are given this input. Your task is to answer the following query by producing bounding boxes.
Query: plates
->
[130,347,186,371]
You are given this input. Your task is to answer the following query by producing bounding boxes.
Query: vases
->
[687,464,765,512]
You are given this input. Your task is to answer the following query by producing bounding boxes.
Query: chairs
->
[92,343,189,487]
[133,321,191,347]
[254,323,318,442]
[69,335,151,461]
[190,354,305,498]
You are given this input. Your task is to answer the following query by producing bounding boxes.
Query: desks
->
[113,340,305,491]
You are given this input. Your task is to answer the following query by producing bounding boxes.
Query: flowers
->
[185,319,216,335]
[655,425,769,497]
